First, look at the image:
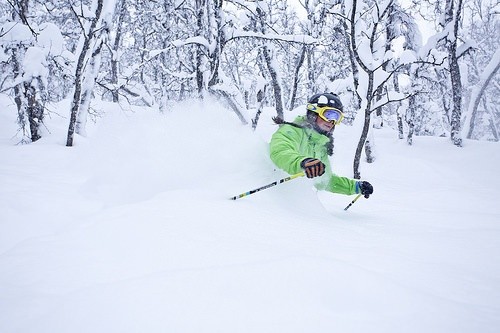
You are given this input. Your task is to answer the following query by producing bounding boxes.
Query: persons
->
[269,92,373,198]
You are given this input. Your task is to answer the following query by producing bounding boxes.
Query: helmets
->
[306,92,345,122]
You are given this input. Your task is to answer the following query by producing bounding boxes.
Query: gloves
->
[358,181,373,199]
[300,158,325,178]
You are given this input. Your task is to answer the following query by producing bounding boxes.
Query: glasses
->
[306,103,344,125]
